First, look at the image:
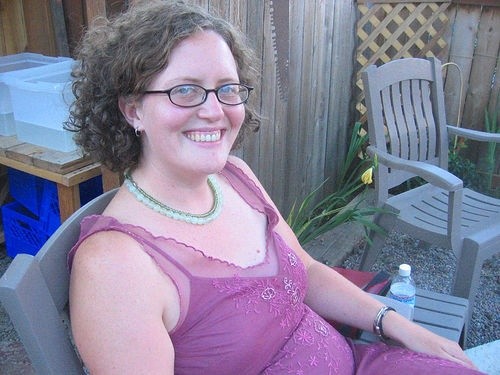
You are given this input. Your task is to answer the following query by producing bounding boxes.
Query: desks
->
[0,140,125,226]
[358,278,471,357]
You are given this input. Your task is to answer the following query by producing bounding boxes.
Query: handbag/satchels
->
[320,267,392,340]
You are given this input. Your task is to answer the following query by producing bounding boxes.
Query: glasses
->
[139,83,254,108]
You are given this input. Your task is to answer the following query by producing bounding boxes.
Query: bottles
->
[389,263,417,321]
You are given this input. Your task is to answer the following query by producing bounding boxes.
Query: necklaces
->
[120,166,224,224]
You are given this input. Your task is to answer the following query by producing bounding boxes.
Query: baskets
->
[0,169,103,258]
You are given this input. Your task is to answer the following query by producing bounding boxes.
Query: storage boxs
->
[0,52,89,152]
[0,167,103,261]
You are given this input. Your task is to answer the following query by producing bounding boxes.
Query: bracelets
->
[374,307,397,343]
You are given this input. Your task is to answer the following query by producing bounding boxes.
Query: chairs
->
[361,57,500,328]
[0,186,128,373]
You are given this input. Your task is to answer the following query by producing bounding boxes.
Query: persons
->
[61,0,486,375]
